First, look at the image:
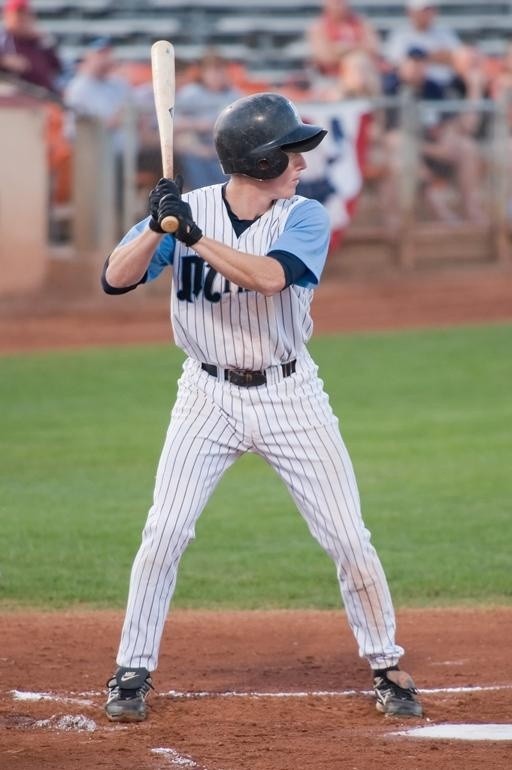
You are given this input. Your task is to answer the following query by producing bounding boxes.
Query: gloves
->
[148,174,202,247]
[198,359,295,387]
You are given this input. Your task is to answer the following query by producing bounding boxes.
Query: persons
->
[3,0,510,247]
[99,92,428,721]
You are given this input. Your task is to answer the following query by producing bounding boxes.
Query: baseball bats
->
[149,39,181,235]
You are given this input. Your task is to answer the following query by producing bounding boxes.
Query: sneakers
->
[373,664,423,719]
[105,665,156,722]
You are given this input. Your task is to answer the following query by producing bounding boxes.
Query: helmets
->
[213,94,328,181]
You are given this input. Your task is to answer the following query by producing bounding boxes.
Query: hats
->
[282,130,329,153]
